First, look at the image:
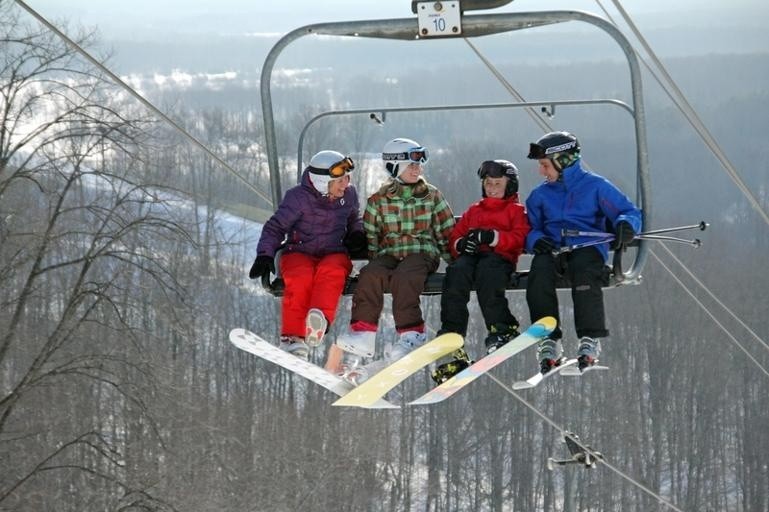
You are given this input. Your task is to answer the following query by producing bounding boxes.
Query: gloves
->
[457,239,478,256]
[467,228,494,245]
[346,232,367,251]
[372,255,396,268]
[533,237,553,255]
[249,256,275,278]
[616,221,633,244]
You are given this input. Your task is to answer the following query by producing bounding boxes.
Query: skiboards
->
[512,356,609,391]
[229,316,558,409]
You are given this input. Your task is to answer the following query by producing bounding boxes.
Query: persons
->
[247,150,372,359]
[336,139,457,363]
[429,157,530,386]
[523,130,644,373]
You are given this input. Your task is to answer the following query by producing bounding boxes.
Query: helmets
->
[537,131,581,171]
[383,137,422,179]
[309,150,350,196]
[481,160,519,198]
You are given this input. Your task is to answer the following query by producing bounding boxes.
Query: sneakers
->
[578,337,600,359]
[431,348,468,385]
[304,308,325,347]
[485,323,518,347]
[280,337,308,356]
[536,340,562,364]
[395,331,423,353]
[337,330,376,358]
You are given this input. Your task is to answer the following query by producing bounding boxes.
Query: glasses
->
[529,140,576,159]
[477,161,518,179]
[310,157,355,178]
[382,147,429,163]
